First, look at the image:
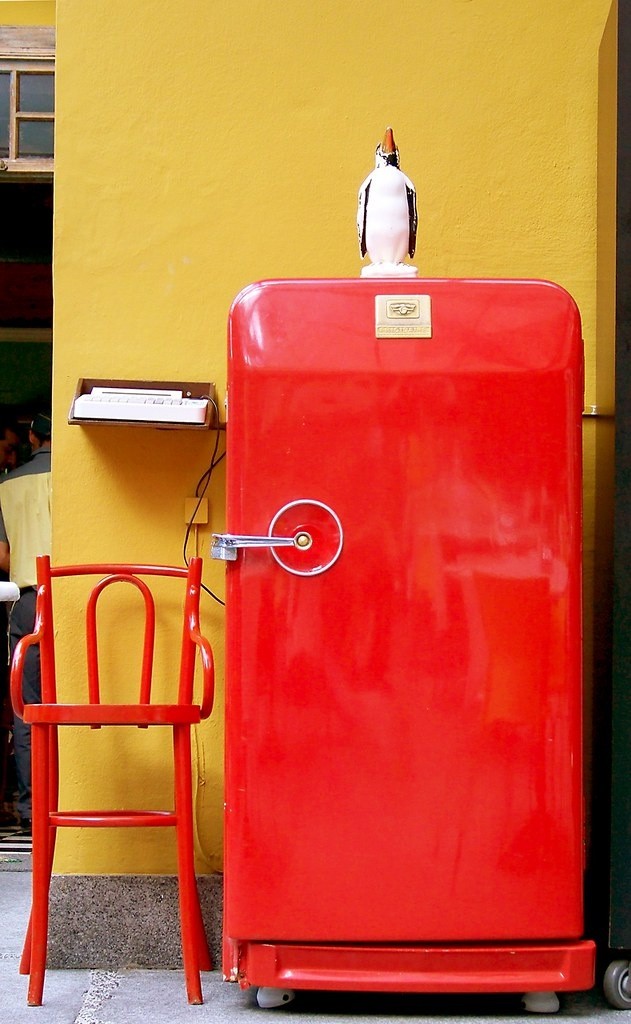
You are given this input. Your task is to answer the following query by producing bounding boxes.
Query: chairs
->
[10,555,213,1005]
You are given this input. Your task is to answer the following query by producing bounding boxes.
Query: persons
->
[0,408,52,835]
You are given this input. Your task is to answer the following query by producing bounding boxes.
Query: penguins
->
[356,126,420,278]
